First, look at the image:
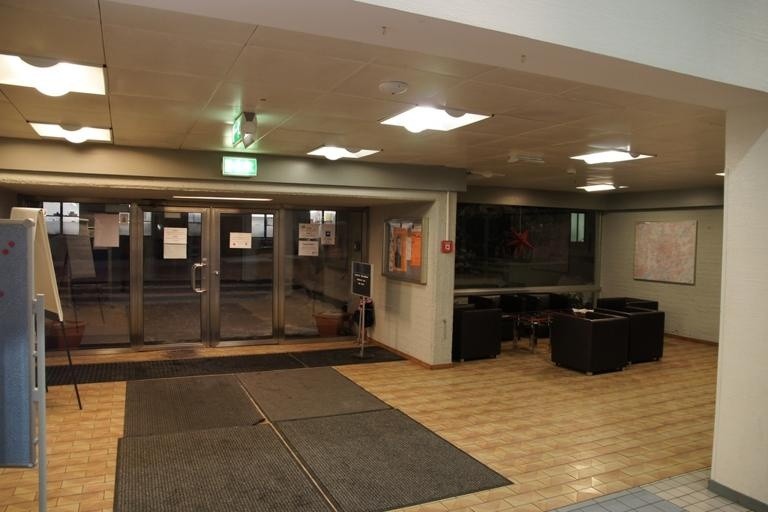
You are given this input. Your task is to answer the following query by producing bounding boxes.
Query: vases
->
[312,311,345,337]
[46,320,87,350]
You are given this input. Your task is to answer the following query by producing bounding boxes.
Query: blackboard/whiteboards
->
[0,219,36,468]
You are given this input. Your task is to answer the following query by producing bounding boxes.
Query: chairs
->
[451,291,666,376]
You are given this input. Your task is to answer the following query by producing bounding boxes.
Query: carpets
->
[271,408,518,512]
[112,422,336,511]
[236,365,395,422]
[121,373,266,437]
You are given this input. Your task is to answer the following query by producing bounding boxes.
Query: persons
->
[395,235,401,269]
